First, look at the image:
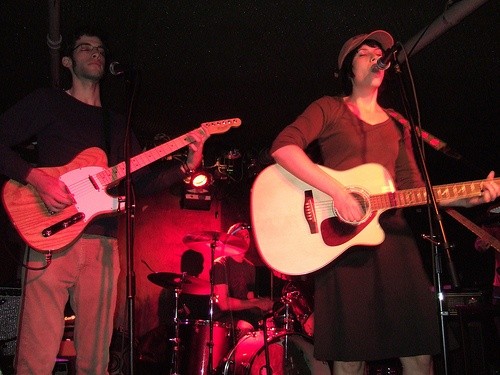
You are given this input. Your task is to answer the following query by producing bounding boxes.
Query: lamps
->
[161,149,263,210]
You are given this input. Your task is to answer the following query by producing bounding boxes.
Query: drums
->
[282,281,314,336]
[173,320,236,375]
[223,328,332,375]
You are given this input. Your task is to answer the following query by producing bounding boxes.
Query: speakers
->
[433,317,499,375]
[107,329,130,374]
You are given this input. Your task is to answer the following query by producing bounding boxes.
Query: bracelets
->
[183,161,193,175]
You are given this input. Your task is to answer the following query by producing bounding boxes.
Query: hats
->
[338,31,394,69]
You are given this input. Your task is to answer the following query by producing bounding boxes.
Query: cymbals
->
[147,272,211,295]
[183,231,248,255]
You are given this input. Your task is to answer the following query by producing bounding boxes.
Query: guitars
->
[444,207,499,253]
[2,118,241,254]
[250,164,500,276]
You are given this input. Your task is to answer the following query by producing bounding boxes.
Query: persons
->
[0,26,211,375]
[208,223,274,332]
[269,30,500,375]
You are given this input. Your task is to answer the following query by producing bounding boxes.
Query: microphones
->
[377,41,399,70]
[110,61,138,78]
